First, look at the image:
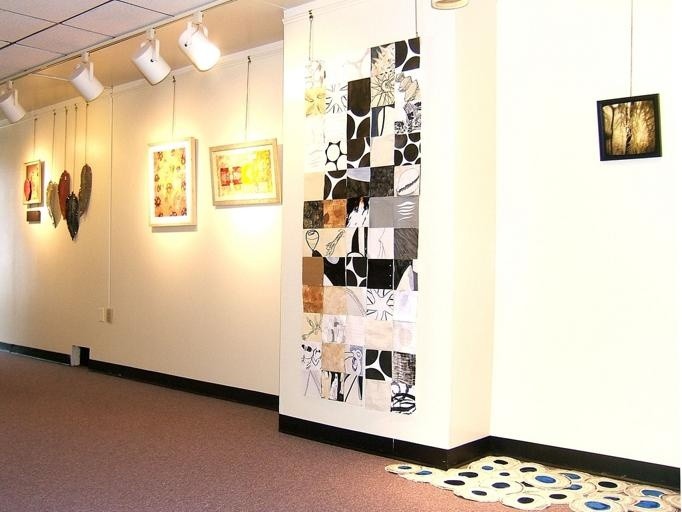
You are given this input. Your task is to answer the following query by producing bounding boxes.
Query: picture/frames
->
[148,137,197,229]
[597,94,663,160]
[209,138,281,206]
[22,160,42,205]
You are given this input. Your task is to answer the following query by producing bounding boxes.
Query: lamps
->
[0,0,236,123]
